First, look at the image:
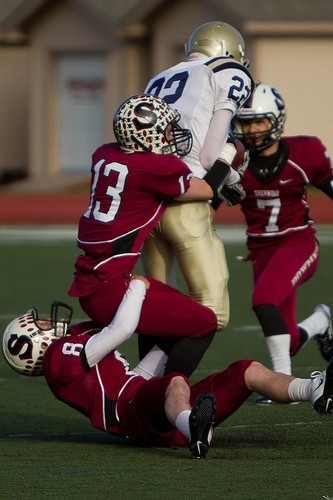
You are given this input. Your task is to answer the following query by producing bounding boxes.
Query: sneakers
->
[255,396,301,406]
[310,358,333,416]
[315,304,333,362]
[189,394,216,457]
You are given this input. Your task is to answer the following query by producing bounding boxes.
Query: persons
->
[66,93,250,378]
[233,82,333,407]
[3,275,333,459]
[132,20,253,377]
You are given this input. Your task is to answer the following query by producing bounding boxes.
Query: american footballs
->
[231,138,246,170]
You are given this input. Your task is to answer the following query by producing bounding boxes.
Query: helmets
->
[188,21,245,67]
[114,94,180,159]
[2,308,68,376]
[231,84,285,153]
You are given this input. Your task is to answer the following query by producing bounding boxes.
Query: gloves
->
[218,185,246,206]
[233,150,250,184]
